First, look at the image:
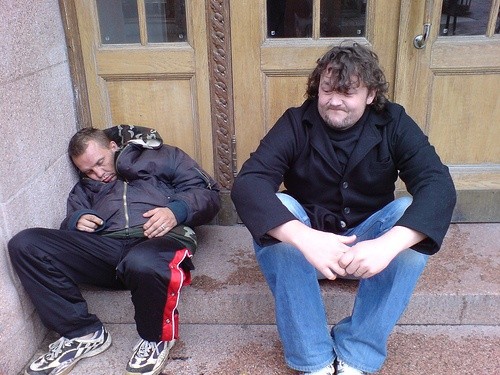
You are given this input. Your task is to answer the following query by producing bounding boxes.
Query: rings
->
[160,224,166,230]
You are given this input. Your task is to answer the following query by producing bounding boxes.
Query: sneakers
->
[125,335,176,374]
[23,326,111,375]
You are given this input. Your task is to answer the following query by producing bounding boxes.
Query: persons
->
[8,122,223,375]
[231,37,457,373]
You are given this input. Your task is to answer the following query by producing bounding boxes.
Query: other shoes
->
[337,357,367,375]
[300,360,336,375]
